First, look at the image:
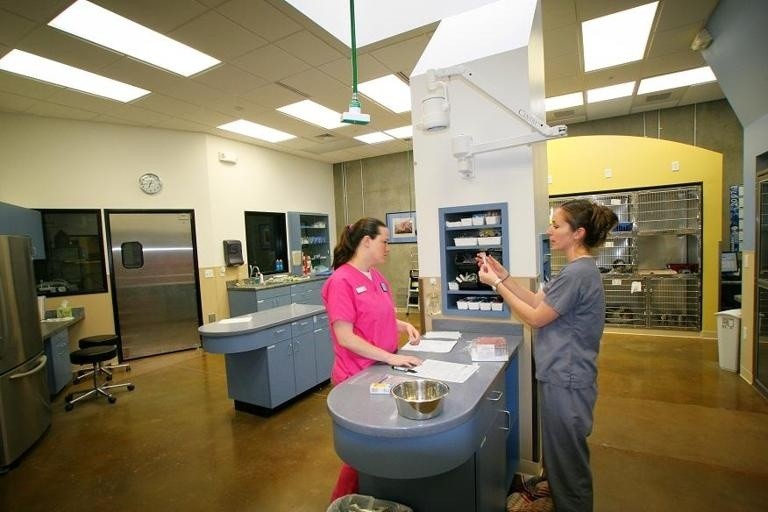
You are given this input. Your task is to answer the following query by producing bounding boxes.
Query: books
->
[468,336,509,362]
[370,374,414,394]
[403,359,481,384]
[402,339,457,354]
[423,330,463,340]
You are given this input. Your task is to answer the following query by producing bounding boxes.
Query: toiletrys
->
[279,259,284,272]
[275,259,280,272]
[307,256,312,273]
[302,255,308,276]
[260,273,264,284]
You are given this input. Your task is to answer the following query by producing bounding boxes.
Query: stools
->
[64,334,136,411]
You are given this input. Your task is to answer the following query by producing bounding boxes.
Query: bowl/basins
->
[391,377,451,420]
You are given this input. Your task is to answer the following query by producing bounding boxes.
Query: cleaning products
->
[275,259,283,271]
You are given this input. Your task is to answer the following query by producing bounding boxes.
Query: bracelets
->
[491,279,503,292]
[501,271,510,281]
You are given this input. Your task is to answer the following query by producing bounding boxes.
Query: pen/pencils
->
[392,364,418,373]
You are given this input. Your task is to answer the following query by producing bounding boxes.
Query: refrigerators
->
[0,234,55,474]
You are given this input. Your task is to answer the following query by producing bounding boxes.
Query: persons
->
[608,259,628,275]
[473,198,620,511]
[320,218,425,504]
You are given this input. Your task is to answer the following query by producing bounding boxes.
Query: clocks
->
[138,173,162,195]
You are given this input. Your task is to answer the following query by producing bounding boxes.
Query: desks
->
[40,306,86,402]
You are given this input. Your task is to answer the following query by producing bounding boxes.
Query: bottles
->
[275,259,283,271]
[302,255,312,276]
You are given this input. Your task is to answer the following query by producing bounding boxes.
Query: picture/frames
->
[385,210,418,245]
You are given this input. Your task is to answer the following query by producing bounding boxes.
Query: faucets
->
[250,265,260,277]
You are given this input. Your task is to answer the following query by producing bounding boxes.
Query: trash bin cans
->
[325,492,417,512]
[713,308,742,373]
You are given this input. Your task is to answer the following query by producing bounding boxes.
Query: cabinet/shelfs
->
[0,201,46,260]
[438,202,511,319]
[200,274,335,418]
[44,327,74,394]
[287,211,332,273]
[326,317,524,512]
[548,181,703,337]
